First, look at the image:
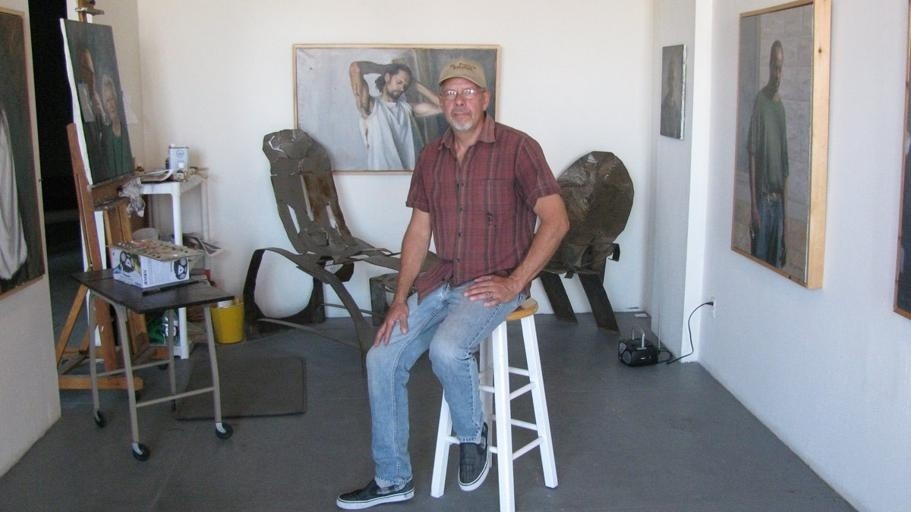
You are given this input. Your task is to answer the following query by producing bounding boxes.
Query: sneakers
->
[337,476,417,510]
[456,422,492,491]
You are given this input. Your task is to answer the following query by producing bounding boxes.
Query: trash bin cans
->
[208,300,245,345]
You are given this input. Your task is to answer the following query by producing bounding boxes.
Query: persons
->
[662,62,678,137]
[76,50,133,180]
[748,41,788,270]
[349,60,441,172]
[336,58,571,510]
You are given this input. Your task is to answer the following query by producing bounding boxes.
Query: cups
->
[134,227,160,244]
[211,304,246,343]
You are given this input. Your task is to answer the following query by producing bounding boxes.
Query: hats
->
[439,57,487,89]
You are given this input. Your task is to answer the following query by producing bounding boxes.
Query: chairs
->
[524,148,637,334]
[240,123,437,373]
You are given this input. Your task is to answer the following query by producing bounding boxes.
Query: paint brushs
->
[141,278,199,297]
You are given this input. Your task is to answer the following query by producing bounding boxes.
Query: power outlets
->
[707,297,716,321]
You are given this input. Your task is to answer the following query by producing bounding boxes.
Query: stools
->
[430,297,559,512]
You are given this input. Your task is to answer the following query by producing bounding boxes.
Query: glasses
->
[440,87,482,100]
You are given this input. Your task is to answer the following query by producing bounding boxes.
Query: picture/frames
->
[290,39,501,179]
[728,0,837,292]
[0,5,46,303]
[892,0,910,321]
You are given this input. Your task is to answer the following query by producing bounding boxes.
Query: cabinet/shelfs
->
[133,171,216,366]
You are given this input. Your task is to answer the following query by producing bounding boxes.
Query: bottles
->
[161,309,182,346]
